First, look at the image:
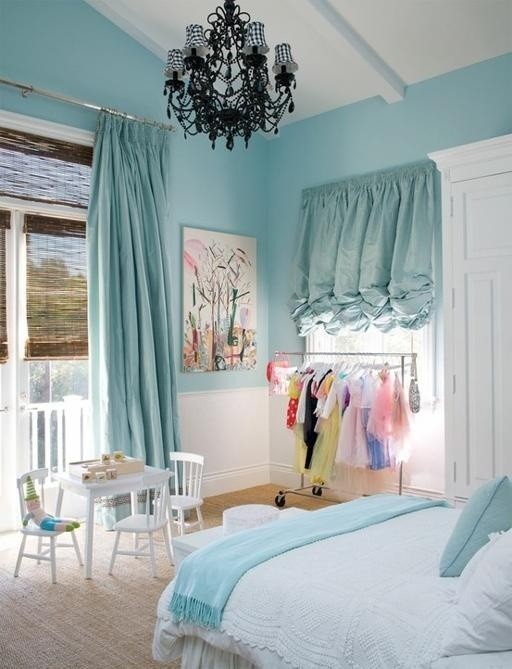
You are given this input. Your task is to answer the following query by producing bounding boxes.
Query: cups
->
[113,451,125,463]
[101,453,112,464]
[81,468,118,484]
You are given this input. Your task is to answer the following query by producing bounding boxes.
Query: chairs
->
[12,449,206,585]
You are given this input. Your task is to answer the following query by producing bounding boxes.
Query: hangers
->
[291,356,400,384]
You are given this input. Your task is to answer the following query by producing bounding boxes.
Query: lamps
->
[160,2,299,150]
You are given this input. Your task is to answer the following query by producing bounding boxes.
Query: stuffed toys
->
[22,476,81,532]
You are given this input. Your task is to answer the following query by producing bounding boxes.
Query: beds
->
[153,473,511,667]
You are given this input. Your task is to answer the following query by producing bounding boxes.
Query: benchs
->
[172,504,319,580]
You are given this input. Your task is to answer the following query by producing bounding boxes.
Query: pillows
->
[434,474,511,664]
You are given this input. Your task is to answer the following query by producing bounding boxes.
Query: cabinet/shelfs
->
[427,133,512,503]
